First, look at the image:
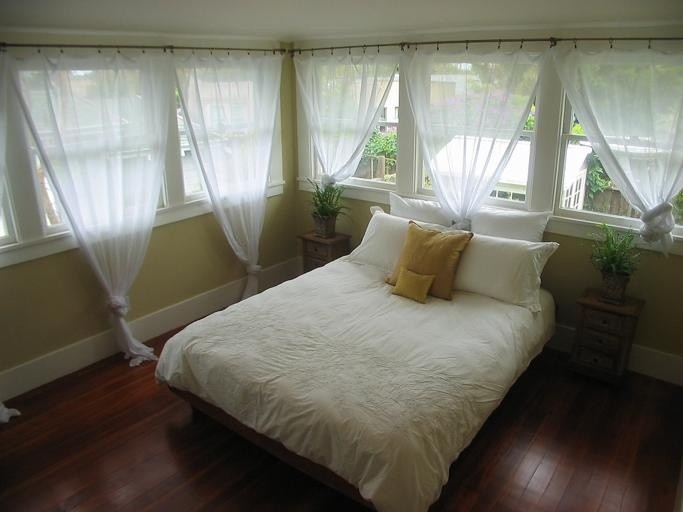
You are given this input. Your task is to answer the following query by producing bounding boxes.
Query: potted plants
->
[589,224,640,306]
[305,178,351,238]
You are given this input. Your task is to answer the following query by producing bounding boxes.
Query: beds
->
[154,190,559,511]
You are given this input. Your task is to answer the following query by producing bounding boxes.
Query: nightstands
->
[567,291,645,392]
[294,229,351,273]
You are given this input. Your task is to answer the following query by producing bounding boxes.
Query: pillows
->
[344,190,558,314]
